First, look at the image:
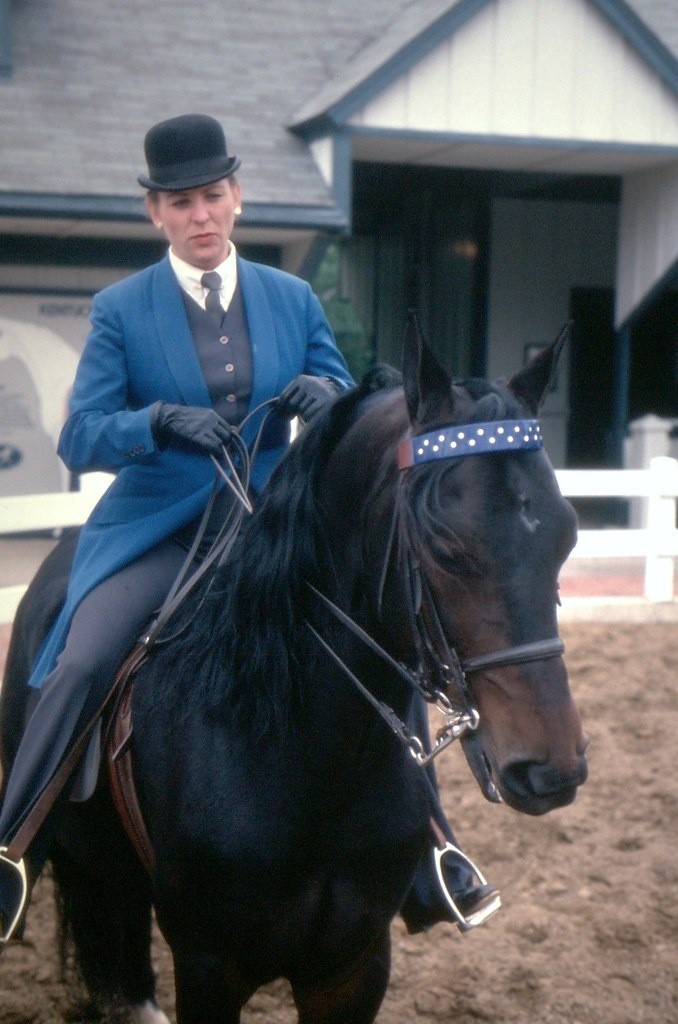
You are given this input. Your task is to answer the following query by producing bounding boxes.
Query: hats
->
[138,114,241,192]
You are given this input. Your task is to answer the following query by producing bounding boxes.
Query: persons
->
[0,114,500,942]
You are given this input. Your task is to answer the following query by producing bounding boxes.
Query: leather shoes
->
[0,869,33,942]
[407,883,500,934]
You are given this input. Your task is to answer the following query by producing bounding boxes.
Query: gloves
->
[152,401,233,458]
[270,374,342,422]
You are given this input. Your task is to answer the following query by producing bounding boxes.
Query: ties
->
[201,271,226,329]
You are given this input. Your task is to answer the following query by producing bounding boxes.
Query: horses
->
[0,310,594,1023]
